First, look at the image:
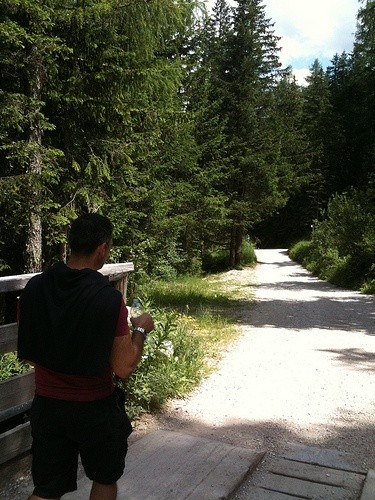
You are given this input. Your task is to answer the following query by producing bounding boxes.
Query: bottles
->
[130,299,143,319]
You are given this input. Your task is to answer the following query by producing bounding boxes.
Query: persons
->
[16,214,154,500]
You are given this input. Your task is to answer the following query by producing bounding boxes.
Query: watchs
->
[133,327,147,340]
